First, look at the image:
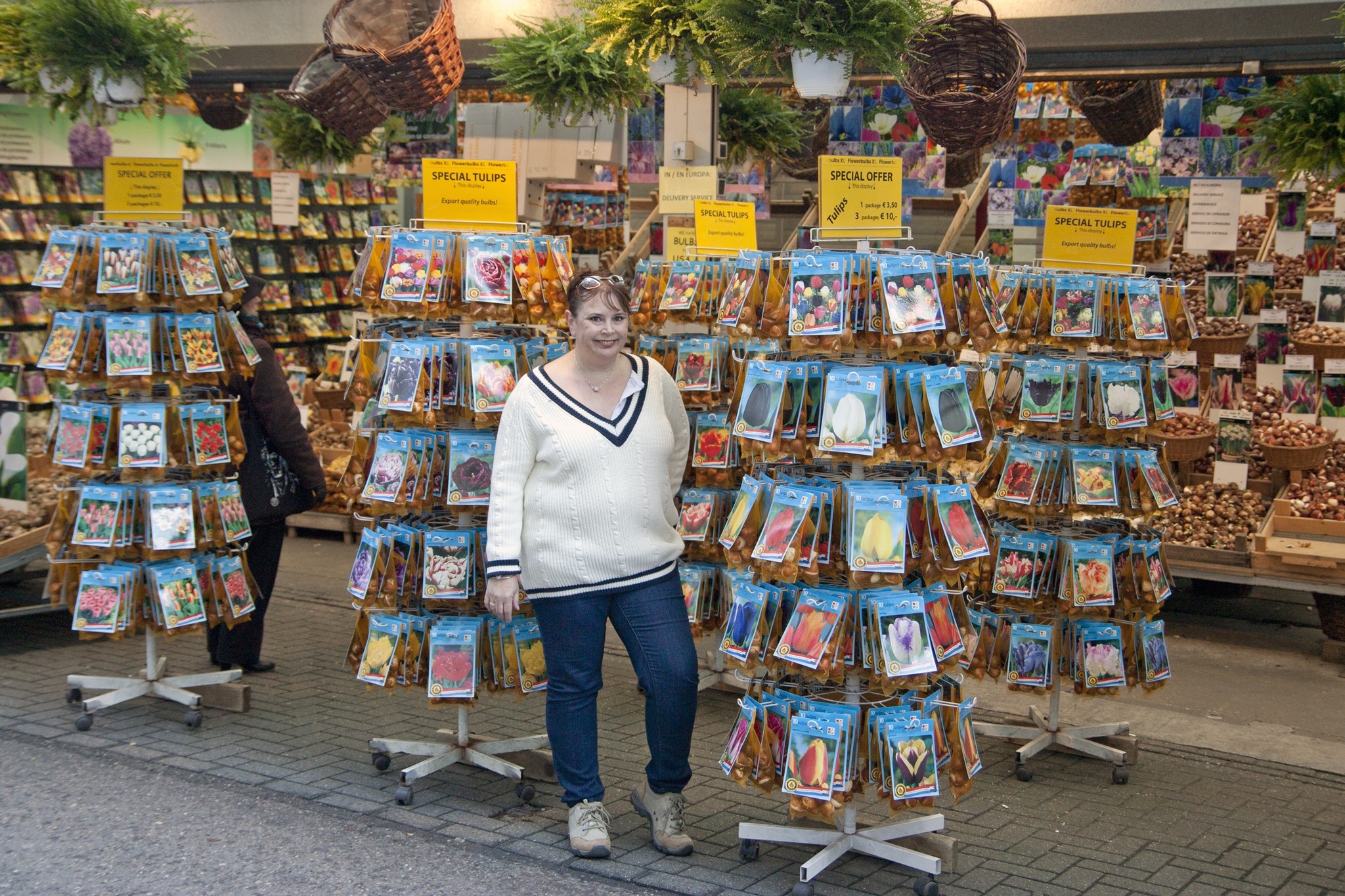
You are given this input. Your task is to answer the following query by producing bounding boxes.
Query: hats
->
[217,252,265,309]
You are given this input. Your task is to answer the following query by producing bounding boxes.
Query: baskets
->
[324,1,465,112]
[190,92,251,129]
[1255,439,1332,470]
[1291,334,1345,370]
[1190,332,1252,363]
[1067,79,1163,147]
[899,0,1026,150]
[1147,429,1217,461]
[273,45,389,140]
[314,389,353,409]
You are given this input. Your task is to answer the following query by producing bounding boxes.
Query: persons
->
[482,270,699,861]
[206,274,330,675]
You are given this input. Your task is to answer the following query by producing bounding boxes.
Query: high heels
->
[219,652,276,672]
[210,652,220,664]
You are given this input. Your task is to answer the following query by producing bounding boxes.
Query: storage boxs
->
[1135,410,1344,582]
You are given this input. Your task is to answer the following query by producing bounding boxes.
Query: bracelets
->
[492,573,517,580]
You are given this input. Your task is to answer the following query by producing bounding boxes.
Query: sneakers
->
[630,778,694,855]
[569,799,611,857]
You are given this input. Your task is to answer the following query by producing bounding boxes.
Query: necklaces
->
[574,348,619,393]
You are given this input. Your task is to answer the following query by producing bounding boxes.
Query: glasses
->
[570,275,627,304]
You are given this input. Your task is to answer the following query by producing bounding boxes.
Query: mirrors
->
[711,80,1344,299]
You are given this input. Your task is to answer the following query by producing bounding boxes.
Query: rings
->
[487,600,497,607]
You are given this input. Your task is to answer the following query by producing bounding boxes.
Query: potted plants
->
[679,1,964,100]
[1,0,228,115]
[559,2,749,90]
[481,14,667,130]
[0,15,117,131]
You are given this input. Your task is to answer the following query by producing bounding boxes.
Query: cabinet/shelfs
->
[0,163,405,630]
[1064,140,1343,300]
[1168,281,1345,419]
[1159,562,1344,642]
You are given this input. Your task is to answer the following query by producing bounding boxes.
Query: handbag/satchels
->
[254,449,315,517]
